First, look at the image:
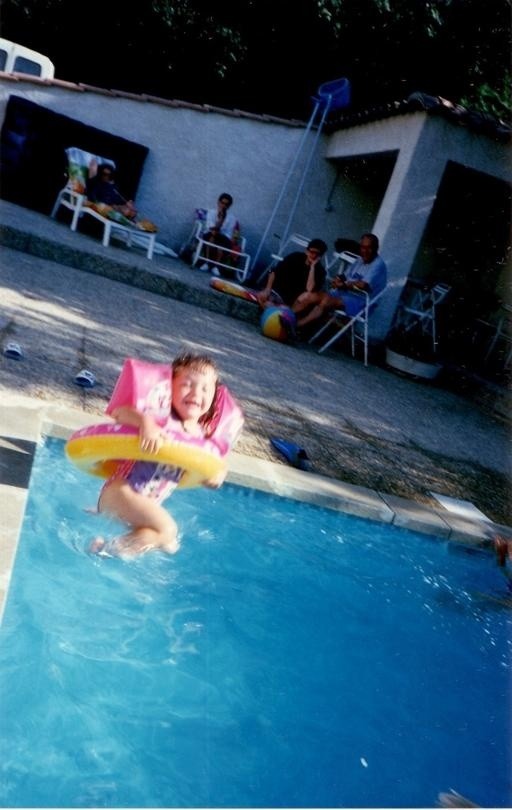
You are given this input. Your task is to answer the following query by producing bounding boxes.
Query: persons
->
[197,194,238,276]
[88,354,228,558]
[290,233,389,335]
[494,534,512,592]
[84,159,139,221]
[255,238,328,311]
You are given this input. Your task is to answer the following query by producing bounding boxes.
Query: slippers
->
[72,368,97,388]
[269,436,313,471]
[3,341,24,361]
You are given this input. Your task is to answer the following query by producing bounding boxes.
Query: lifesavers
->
[106,359,245,457]
[66,424,228,488]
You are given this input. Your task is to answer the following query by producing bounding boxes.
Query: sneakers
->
[200,261,220,276]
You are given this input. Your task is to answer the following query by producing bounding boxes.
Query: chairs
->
[182,208,249,282]
[257,234,451,368]
[485,302,512,368]
[51,147,156,259]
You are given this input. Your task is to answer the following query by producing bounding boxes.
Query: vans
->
[0,38,55,80]
